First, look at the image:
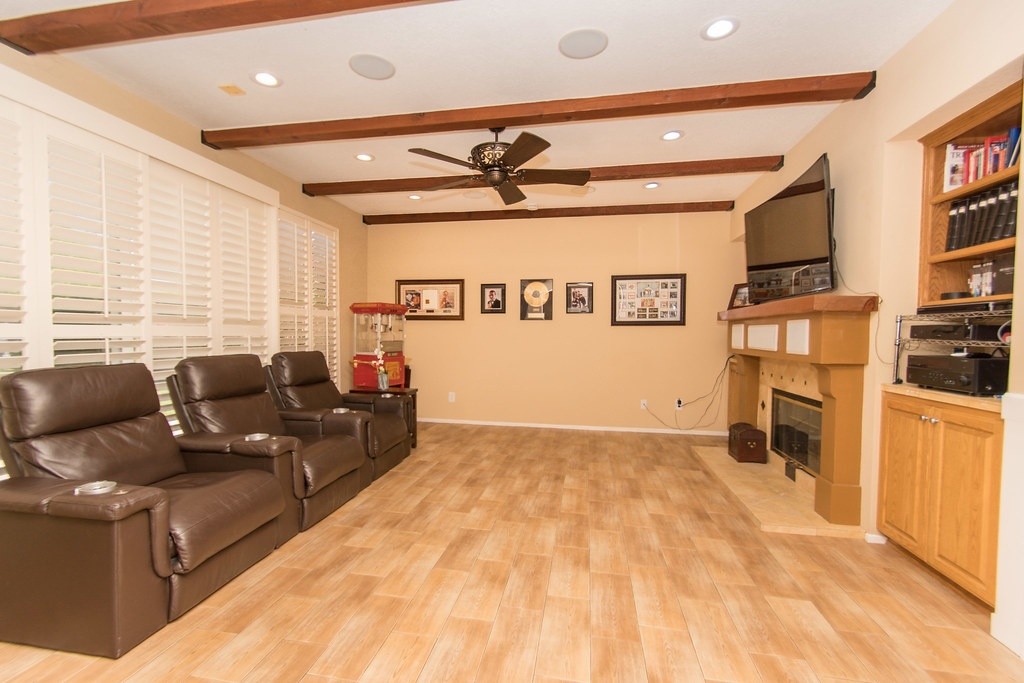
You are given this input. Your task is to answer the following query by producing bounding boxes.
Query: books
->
[943,127,1021,252]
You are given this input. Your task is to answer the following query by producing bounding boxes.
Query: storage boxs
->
[729,422,766,464]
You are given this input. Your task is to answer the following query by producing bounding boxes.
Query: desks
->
[351,384,418,447]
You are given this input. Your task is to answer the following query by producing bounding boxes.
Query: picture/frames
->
[728,283,760,310]
[611,273,687,325]
[481,283,506,313]
[566,283,593,313]
[395,279,465,320]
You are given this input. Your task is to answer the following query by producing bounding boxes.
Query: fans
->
[409,125,590,207]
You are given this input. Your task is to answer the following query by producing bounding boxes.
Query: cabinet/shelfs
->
[883,393,1004,608]
[916,80,1024,311]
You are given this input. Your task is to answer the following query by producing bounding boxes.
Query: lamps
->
[470,126,513,189]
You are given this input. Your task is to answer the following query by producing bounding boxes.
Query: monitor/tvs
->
[744,152,838,304]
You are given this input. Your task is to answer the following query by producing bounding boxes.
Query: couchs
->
[165,354,373,529]
[0,364,305,660]
[268,349,411,479]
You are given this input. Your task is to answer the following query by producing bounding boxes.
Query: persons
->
[487,290,501,307]
[441,291,453,308]
[572,289,587,307]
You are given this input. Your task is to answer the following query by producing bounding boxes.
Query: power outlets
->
[640,399,648,409]
[675,400,683,411]
